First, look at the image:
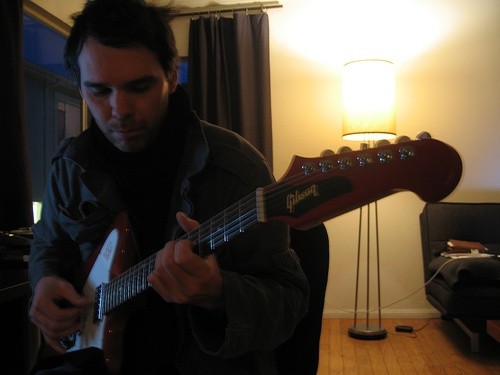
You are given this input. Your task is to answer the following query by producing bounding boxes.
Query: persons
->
[26,0,308,375]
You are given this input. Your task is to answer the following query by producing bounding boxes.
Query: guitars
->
[39,130,463,372]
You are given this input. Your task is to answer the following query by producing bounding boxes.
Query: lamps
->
[340,58,400,341]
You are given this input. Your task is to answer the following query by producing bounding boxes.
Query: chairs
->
[419,202,500,353]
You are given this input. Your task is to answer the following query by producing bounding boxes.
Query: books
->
[447,239,486,253]
